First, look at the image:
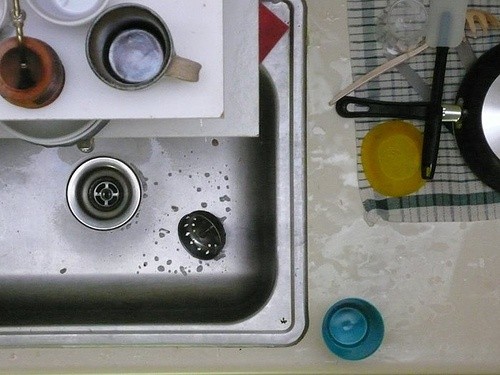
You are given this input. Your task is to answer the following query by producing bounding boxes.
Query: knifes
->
[379,47,456,135]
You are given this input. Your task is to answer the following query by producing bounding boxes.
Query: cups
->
[84,2,203,92]
[320,298,385,362]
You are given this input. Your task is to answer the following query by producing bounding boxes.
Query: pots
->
[334,41,500,192]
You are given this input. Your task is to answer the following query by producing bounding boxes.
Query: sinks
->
[0,64,289,334]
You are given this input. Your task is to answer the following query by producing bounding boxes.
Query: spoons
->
[10,0,37,88]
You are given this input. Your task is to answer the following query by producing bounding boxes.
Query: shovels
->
[421,0,467,178]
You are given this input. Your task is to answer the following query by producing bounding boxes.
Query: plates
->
[1,1,8,27]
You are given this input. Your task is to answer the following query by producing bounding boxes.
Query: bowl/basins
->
[360,121,432,199]
[26,0,107,26]
